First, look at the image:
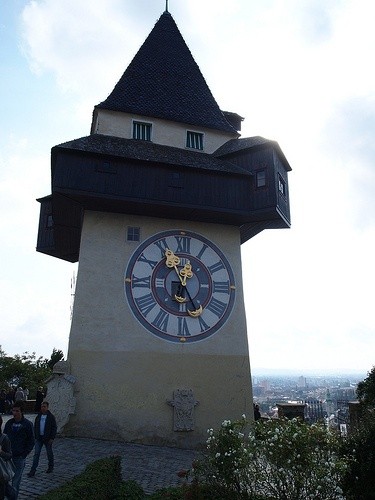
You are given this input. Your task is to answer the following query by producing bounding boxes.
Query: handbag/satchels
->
[0,457,16,483]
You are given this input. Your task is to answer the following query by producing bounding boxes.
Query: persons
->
[0,413,14,500]
[3,402,36,497]
[26,401,57,477]
[35,386,44,413]
[0,386,29,414]
[253,402,261,420]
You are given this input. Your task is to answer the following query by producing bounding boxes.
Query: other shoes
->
[47,468,52,473]
[27,471,35,476]
